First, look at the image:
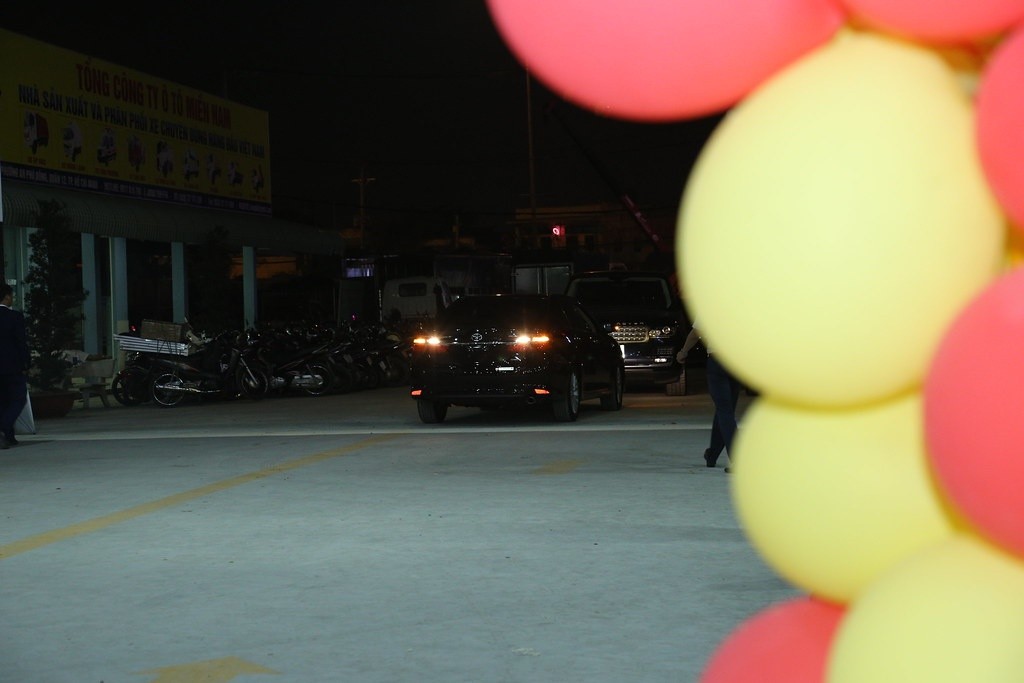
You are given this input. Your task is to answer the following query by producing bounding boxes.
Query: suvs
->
[566,270,687,397]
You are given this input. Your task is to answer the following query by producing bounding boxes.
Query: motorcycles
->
[105,308,431,411]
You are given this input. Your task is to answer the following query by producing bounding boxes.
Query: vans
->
[382,278,443,324]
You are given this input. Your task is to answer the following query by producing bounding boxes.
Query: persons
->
[0,280,28,450]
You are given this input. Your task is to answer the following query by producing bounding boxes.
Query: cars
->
[410,292,626,425]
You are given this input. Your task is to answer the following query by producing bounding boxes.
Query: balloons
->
[488,2,1024,683]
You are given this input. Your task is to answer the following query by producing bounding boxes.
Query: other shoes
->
[703,448,718,467]
[0,428,19,449]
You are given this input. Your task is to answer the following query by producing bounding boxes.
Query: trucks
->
[512,263,577,300]
